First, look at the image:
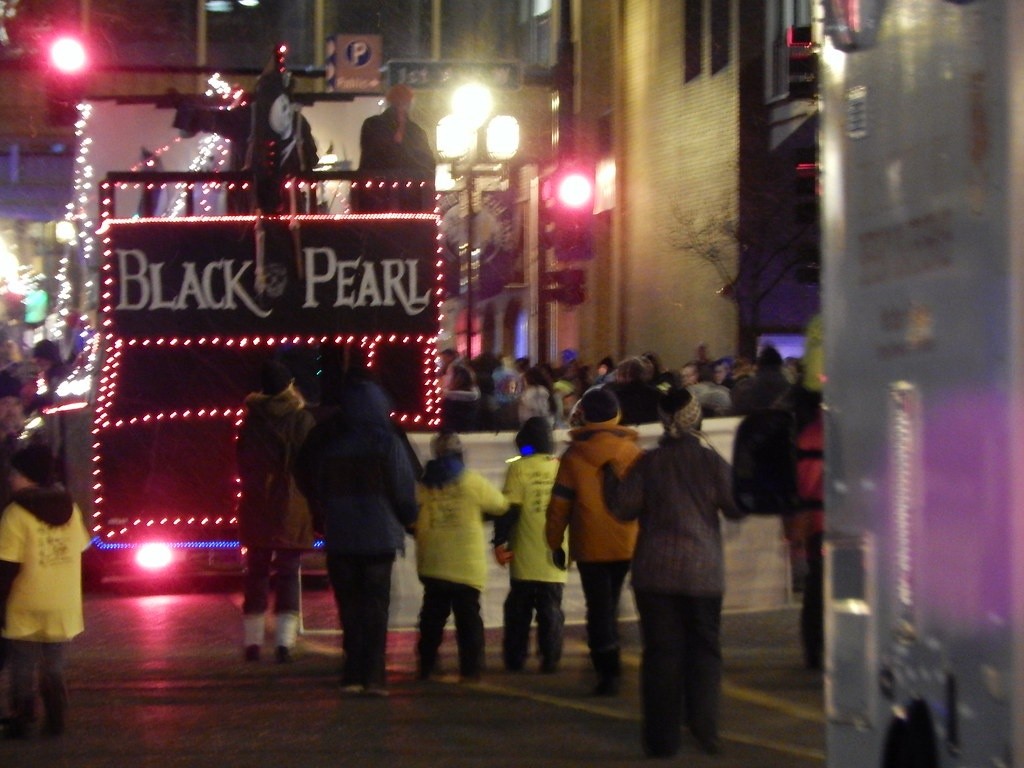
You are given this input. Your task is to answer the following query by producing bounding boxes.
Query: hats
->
[263,358,292,394]
[11,444,56,486]
[515,417,555,454]
[338,362,375,400]
[0,369,22,399]
[436,433,465,456]
[657,388,702,436]
[579,389,621,426]
[34,340,59,362]
[388,84,412,104]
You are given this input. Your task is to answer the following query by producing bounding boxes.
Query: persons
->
[600,383,751,755]
[0,337,75,504]
[293,364,422,694]
[414,430,514,680]
[797,417,826,673]
[769,356,824,568]
[234,357,317,664]
[350,84,437,213]
[165,86,318,216]
[544,385,644,699]
[0,440,91,738]
[495,414,574,678]
[437,339,783,427]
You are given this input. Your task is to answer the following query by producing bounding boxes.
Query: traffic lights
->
[550,269,584,305]
[40,34,83,137]
[541,170,591,253]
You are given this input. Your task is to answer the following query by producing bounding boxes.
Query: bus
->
[84,169,443,584]
[812,0,1024,768]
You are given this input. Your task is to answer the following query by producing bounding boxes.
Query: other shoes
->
[0,721,30,739]
[276,645,288,659]
[43,717,65,733]
[338,672,375,695]
[246,645,260,661]
[581,683,618,697]
[702,734,721,752]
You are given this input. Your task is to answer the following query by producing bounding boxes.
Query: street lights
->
[433,81,521,359]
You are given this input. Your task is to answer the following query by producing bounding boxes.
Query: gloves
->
[553,547,566,570]
[495,546,512,566]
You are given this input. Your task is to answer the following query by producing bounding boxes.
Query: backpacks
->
[733,400,825,514]
